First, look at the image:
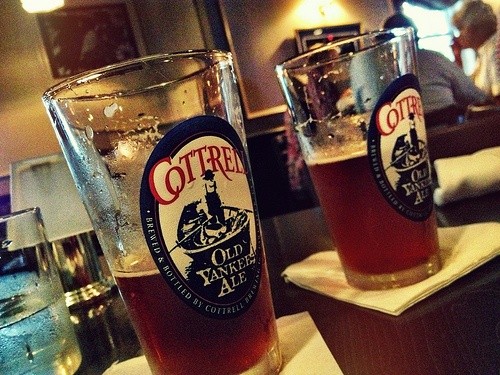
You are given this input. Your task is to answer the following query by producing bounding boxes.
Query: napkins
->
[101,311,344,375]
[432,146,500,205]
[280,221,500,317]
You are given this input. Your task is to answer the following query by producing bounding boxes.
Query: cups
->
[41,47,283,375]
[275,26,443,290]
[0,207,85,375]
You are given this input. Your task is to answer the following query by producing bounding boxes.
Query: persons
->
[349,12,492,121]
[447,0,500,108]
[304,48,349,121]
